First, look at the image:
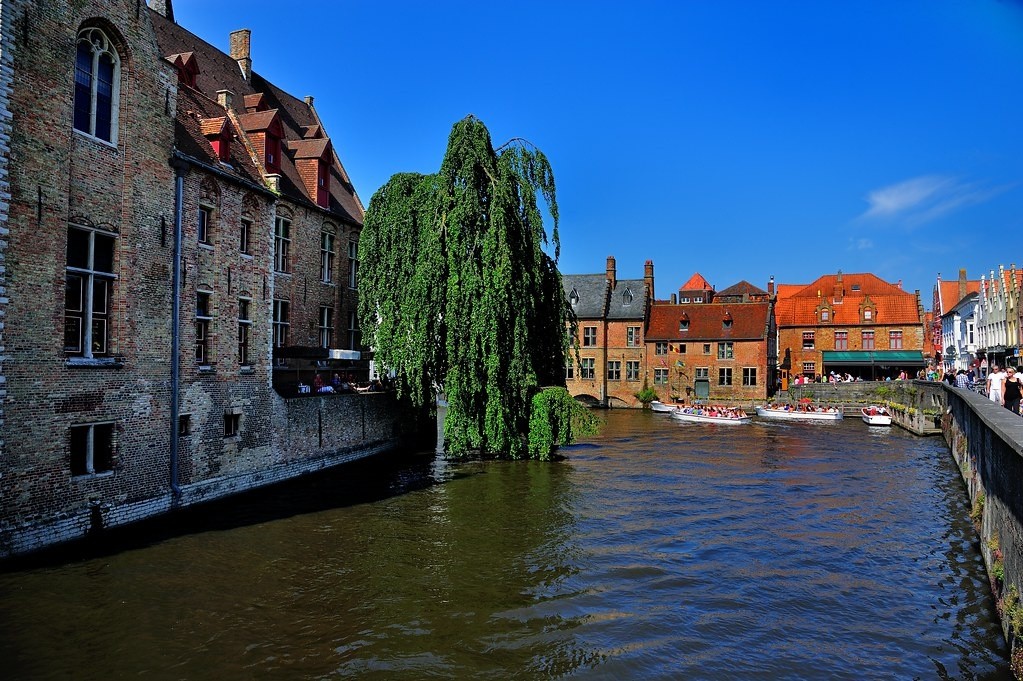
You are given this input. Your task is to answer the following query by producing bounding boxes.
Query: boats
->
[860,405,893,426]
[650,400,739,413]
[670,406,754,425]
[754,403,845,420]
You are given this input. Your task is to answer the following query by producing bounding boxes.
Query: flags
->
[669,344,680,353]
[675,359,685,366]
[661,358,667,366]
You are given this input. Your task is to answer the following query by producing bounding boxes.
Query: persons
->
[767,401,839,412]
[870,404,887,416]
[776,376,782,389]
[876,370,908,381]
[942,366,978,388]
[315,372,340,387]
[789,373,863,384]
[917,363,940,381]
[678,405,742,418]
[987,365,1023,417]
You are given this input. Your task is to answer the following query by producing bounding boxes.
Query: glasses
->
[1007,371,1013,373]
